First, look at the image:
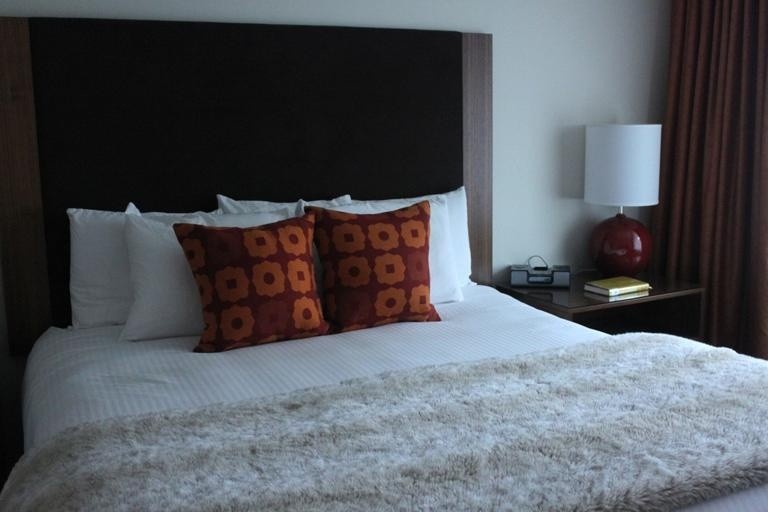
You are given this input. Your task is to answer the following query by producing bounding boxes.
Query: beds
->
[0,16,768,512]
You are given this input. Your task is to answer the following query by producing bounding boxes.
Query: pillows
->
[408,185,477,290]
[296,194,464,308]
[65,204,211,332]
[213,191,355,214]
[169,211,331,352]
[119,201,280,343]
[304,200,443,334]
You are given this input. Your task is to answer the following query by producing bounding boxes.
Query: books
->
[583,290,649,302]
[583,274,650,297]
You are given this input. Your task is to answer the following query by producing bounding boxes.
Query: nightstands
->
[496,268,707,344]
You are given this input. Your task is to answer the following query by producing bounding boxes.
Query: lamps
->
[583,123,664,274]
